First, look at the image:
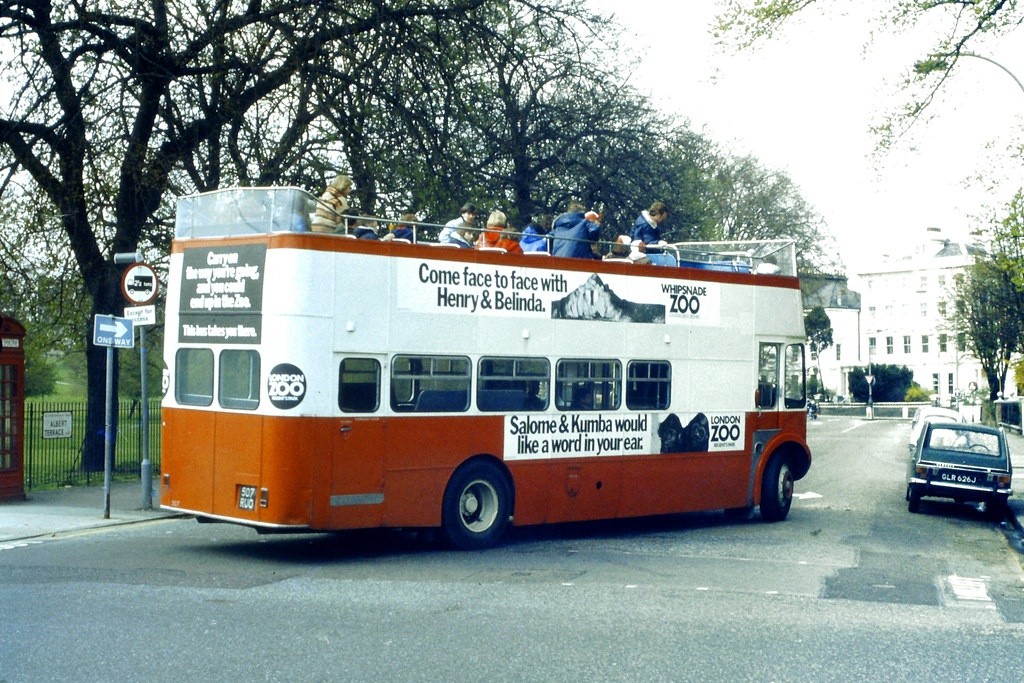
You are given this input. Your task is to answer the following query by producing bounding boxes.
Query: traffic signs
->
[92,313,136,350]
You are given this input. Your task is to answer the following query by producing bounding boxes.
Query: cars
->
[908,405,966,450]
[905,419,1014,522]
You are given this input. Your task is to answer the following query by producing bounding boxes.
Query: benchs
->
[341,381,535,409]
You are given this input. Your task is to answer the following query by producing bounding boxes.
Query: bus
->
[154,184,814,554]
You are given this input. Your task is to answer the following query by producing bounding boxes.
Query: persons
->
[309,175,673,265]
[807,401,818,420]
[567,386,596,411]
[521,379,546,410]
